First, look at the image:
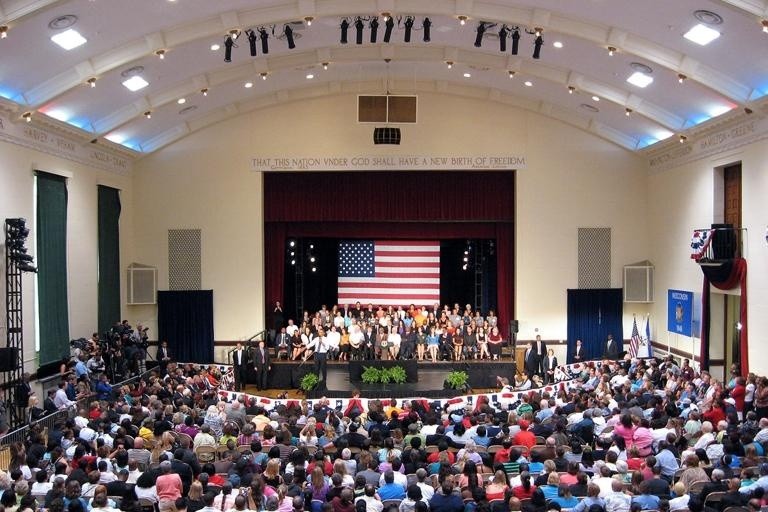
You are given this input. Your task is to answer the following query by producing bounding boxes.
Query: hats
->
[519,420,529,429]
[681,398,691,405]
[724,398,736,407]
[615,460,628,473]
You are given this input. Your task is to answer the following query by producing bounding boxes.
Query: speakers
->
[509,319,518,333]
[623,265,655,303]
[0,348,19,372]
[127,263,158,305]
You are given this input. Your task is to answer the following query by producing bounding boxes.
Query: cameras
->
[242,488,249,497]
[721,479,729,485]
[306,483,312,486]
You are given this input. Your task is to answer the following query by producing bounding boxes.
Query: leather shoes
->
[275,356,501,362]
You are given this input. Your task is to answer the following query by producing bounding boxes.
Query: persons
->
[1,299,768,512]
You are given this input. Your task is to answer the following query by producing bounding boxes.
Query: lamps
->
[340,14,432,45]
[224,21,297,62]
[473,20,544,59]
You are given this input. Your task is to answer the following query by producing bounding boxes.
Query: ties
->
[317,338,322,353]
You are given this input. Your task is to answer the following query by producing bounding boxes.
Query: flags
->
[335,242,442,309]
